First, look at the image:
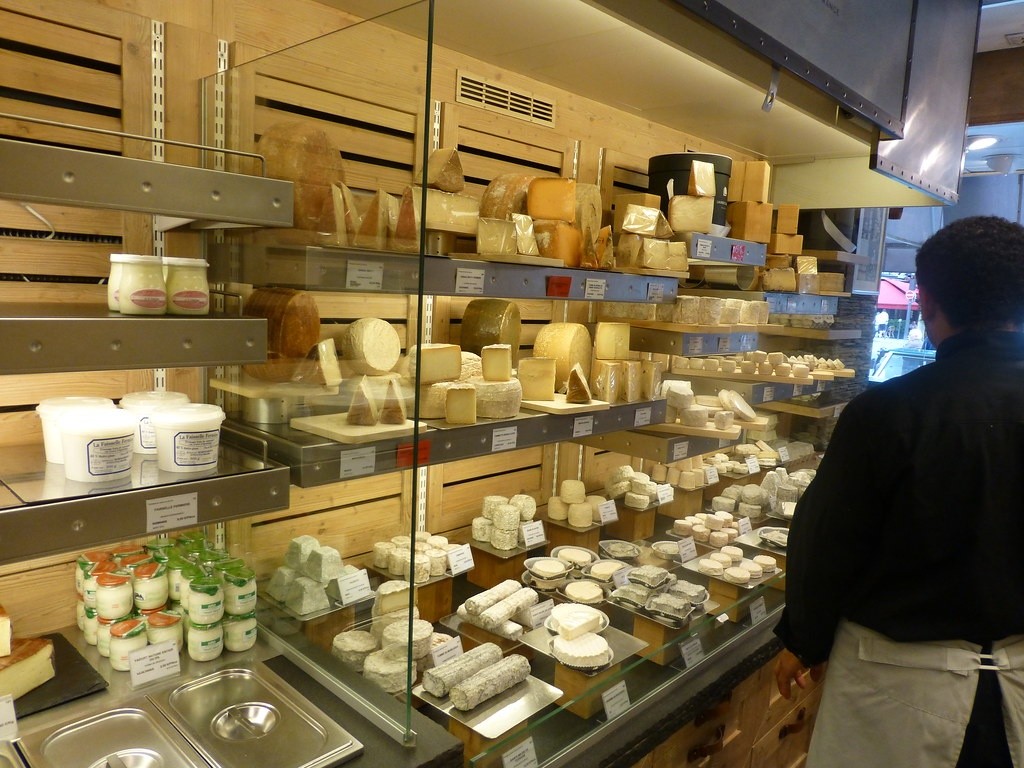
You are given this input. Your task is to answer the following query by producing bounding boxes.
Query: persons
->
[875,308,936,350]
[772,215,1024,768]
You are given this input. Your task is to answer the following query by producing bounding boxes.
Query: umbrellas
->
[876,277,920,310]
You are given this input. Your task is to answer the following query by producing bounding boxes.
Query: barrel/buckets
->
[33,392,227,482]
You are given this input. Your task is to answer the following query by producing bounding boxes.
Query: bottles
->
[109,253,209,315]
[76,530,257,671]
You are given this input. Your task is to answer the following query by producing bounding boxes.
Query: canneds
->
[107,253,211,316]
[74,533,257,672]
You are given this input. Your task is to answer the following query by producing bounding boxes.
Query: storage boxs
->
[298,468,786,768]
[728,161,844,292]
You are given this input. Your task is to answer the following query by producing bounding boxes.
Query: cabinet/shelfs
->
[0,0,981,768]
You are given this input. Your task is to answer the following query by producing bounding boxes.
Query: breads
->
[0,604,55,701]
[244,122,844,709]
